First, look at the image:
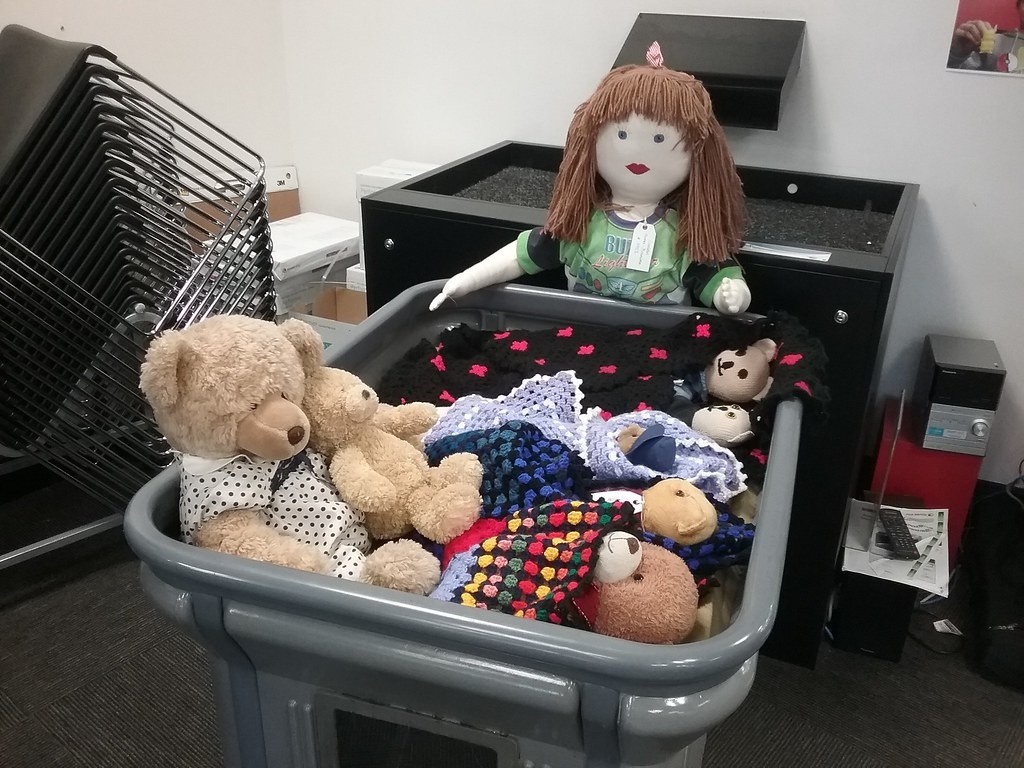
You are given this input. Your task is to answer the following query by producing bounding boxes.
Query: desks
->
[360,139,921,671]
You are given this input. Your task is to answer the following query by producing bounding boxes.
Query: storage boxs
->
[149,159,440,349]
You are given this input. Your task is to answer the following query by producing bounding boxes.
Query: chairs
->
[0,23,279,517]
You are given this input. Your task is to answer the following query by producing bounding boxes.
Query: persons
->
[947,0,1024,74]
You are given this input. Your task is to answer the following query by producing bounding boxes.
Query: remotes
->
[880,508,920,560]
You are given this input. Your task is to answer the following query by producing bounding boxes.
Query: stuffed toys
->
[428,39,751,316]
[138,314,776,643]
